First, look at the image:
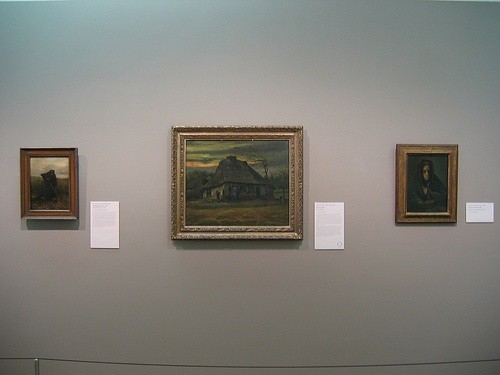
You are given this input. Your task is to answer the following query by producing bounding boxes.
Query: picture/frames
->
[20,148,79,220]
[395,144,458,223]
[169,125,304,241]
